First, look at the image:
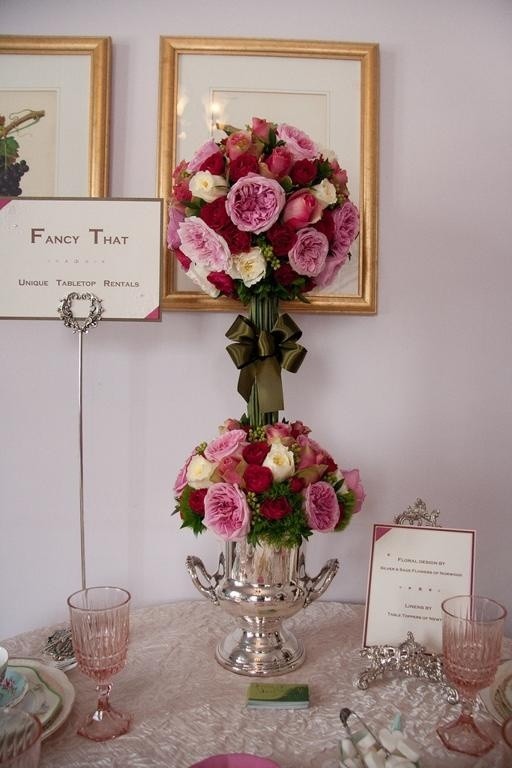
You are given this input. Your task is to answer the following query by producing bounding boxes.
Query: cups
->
[1,646,11,688]
[66,585,132,743]
[436,591,508,757]
[0,707,43,767]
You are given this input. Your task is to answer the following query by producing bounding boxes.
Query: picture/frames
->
[156,35,382,316]
[1,34,114,199]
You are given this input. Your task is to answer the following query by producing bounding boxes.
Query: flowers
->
[170,412,369,550]
[165,116,361,430]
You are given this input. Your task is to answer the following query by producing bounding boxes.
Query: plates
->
[477,658,512,726]
[0,665,62,728]
[0,657,75,749]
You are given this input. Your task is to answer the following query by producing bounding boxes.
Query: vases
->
[184,534,340,677]
